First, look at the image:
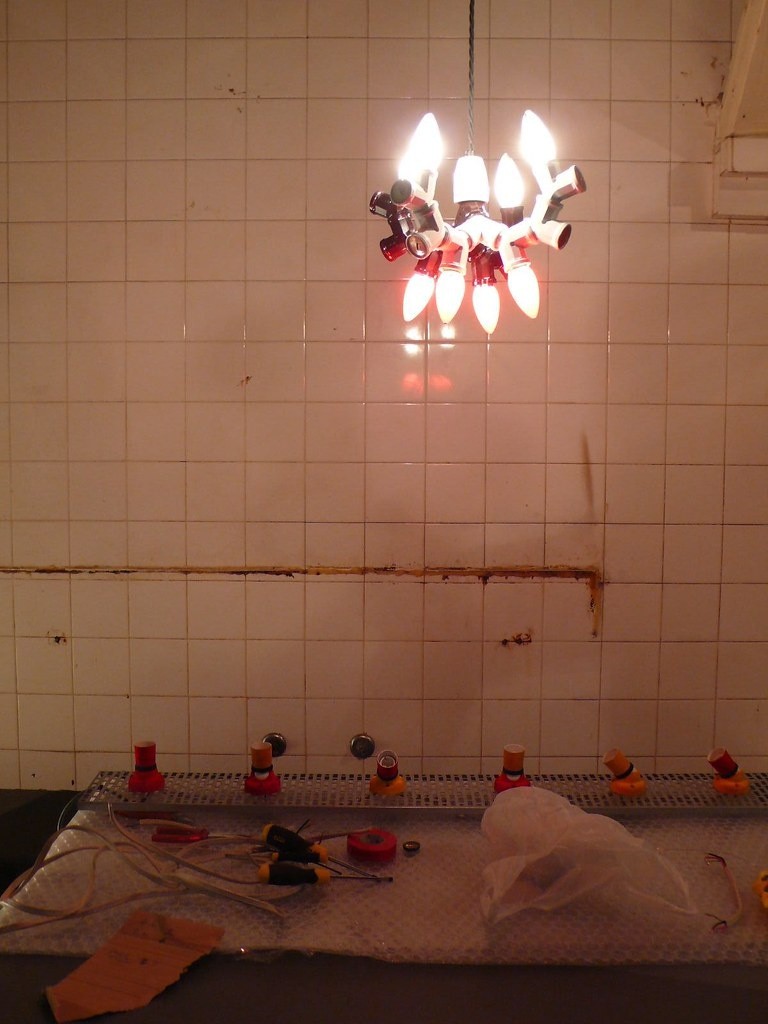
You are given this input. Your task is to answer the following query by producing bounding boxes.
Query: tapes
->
[347,828,397,865]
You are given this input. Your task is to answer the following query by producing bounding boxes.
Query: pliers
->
[152,826,247,843]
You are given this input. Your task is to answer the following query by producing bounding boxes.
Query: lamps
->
[369,750,406,797]
[708,747,749,794]
[493,744,531,795]
[369,0,587,336]
[602,748,646,797]
[128,740,164,793]
[243,742,282,797]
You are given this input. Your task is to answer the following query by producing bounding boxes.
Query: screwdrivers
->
[260,825,383,884]
[259,862,395,885]
